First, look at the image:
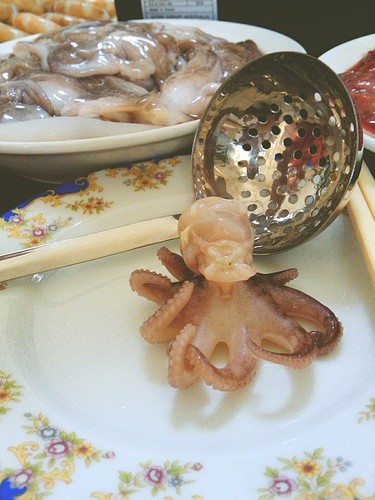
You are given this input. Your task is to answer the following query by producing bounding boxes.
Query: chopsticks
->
[346,161,374,279]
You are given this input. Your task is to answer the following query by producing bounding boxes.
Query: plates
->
[318,32,374,152]
[0,155,375,498]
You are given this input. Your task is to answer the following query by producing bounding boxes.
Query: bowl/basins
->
[0,17,307,185]
